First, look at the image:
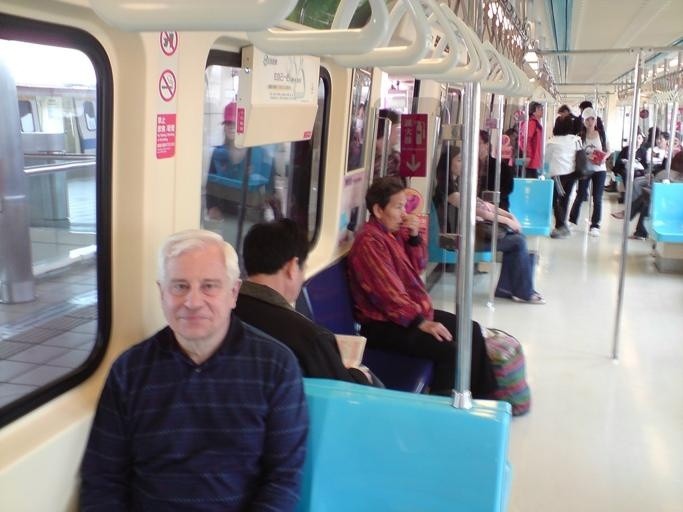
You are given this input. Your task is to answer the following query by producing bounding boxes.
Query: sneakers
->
[628,234,648,241]
[512,291,544,304]
[611,210,625,220]
[551,221,600,238]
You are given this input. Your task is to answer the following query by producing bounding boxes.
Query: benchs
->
[293,372,513,511]
[427,158,557,267]
[610,150,681,273]
[298,251,437,395]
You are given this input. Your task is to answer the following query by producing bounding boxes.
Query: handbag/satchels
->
[482,329,531,416]
[478,219,514,241]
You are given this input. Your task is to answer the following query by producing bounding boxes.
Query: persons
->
[79,226,307,512]
[234,216,385,389]
[205,102,272,280]
[351,105,548,304]
[349,174,498,404]
[603,126,682,240]
[518,100,610,240]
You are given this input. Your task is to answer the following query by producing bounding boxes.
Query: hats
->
[221,103,237,124]
[581,107,597,121]
